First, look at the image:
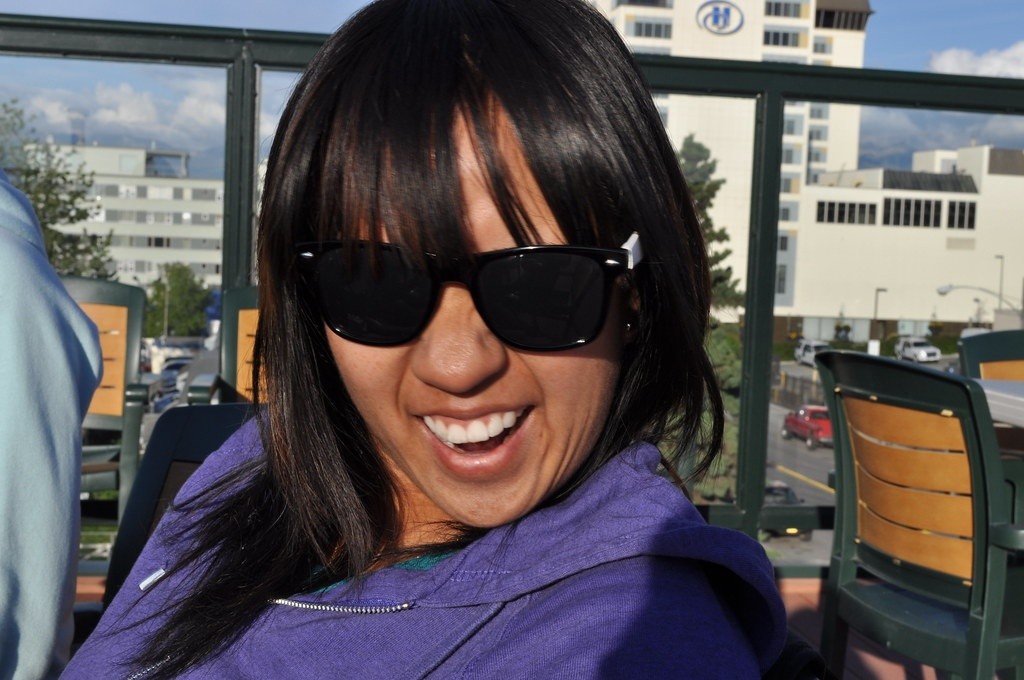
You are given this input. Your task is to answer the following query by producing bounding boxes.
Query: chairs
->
[60,273,144,587]
[812,348,1024,680]
[216,281,266,409]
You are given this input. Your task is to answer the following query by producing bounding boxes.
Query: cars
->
[794,340,833,369]
[152,358,194,413]
[783,405,834,451]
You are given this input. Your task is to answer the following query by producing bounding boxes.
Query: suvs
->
[896,337,942,364]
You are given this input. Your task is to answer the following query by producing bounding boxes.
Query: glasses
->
[294,228,643,348]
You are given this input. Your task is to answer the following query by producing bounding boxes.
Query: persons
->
[48,1,794,679]
[0,164,105,678]
